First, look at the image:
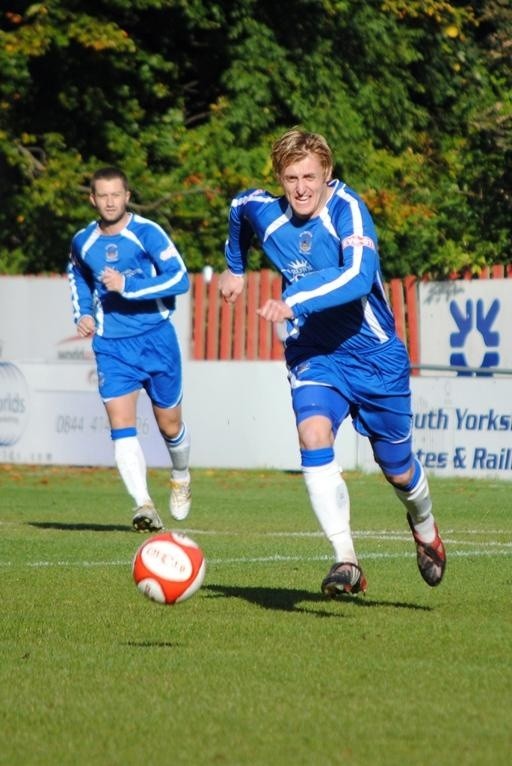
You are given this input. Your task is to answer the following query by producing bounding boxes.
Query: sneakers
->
[407,512,446,585]
[170,472,191,521]
[321,563,366,595]
[133,505,162,533]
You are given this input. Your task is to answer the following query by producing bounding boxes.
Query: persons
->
[212,125,446,601]
[64,165,194,536]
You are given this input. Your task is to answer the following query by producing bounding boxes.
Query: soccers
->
[132,532,206,604]
